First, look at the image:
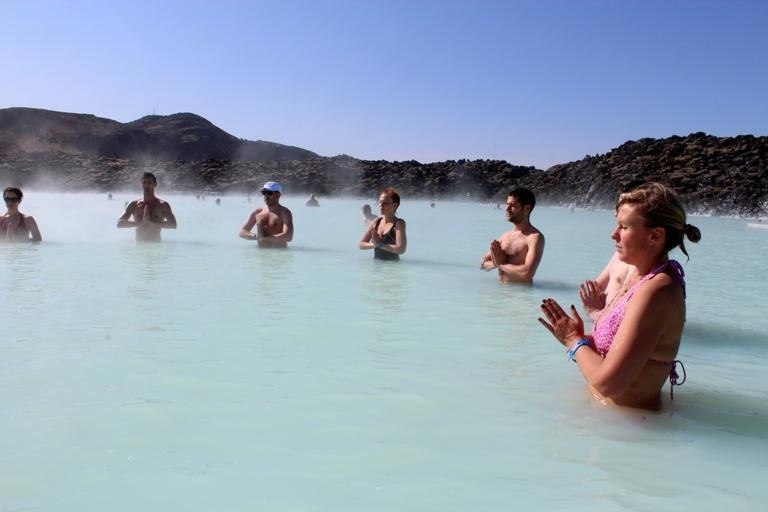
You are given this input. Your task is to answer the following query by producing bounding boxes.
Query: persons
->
[305,194,319,206]
[495,204,501,210]
[117,173,177,240]
[480,188,544,283]
[239,182,293,248]
[196,195,220,205]
[538,181,701,410]
[108,194,112,200]
[358,189,406,261]
[362,205,377,224]
[0,188,42,241]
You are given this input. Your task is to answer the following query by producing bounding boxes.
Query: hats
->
[260,181,282,193]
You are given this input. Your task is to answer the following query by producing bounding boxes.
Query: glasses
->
[4,197,19,203]
[262,190,277,196]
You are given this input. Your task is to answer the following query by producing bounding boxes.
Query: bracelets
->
[379,242,384,248]
[255,234,256,238]
